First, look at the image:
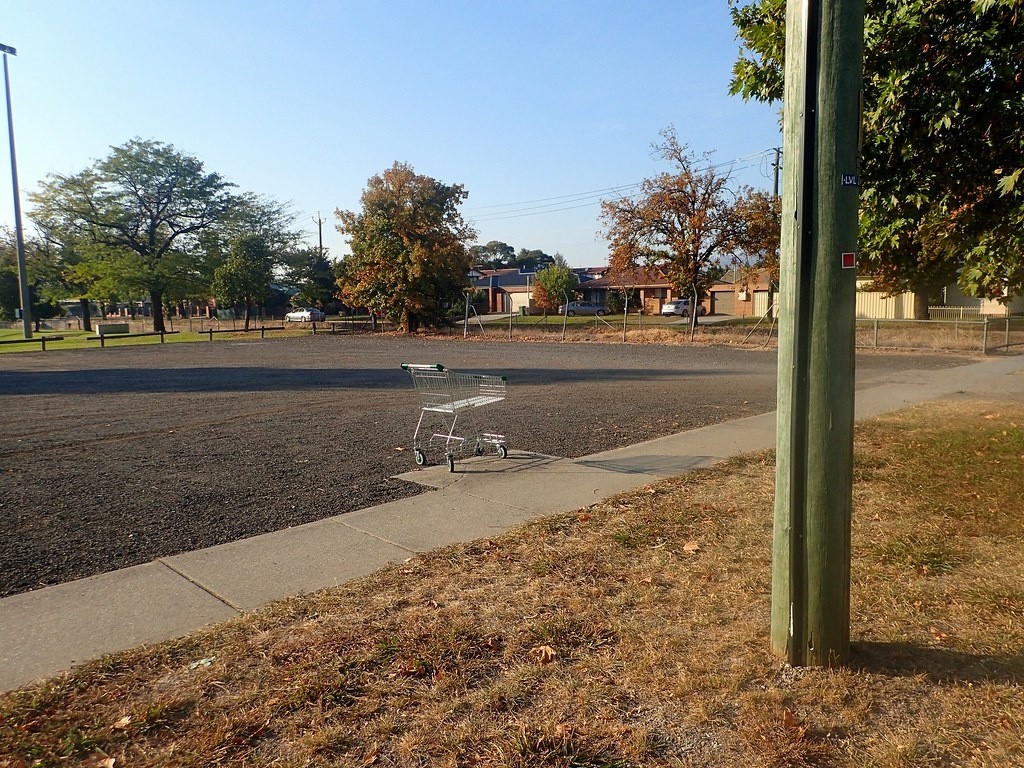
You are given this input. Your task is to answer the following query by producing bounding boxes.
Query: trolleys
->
[399,361,510,475]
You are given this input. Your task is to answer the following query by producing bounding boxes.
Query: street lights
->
[0,42,34,339]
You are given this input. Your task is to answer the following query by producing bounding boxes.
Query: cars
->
[661,299,707,318]
[558,300,610,317]
[285,306,326,323]
